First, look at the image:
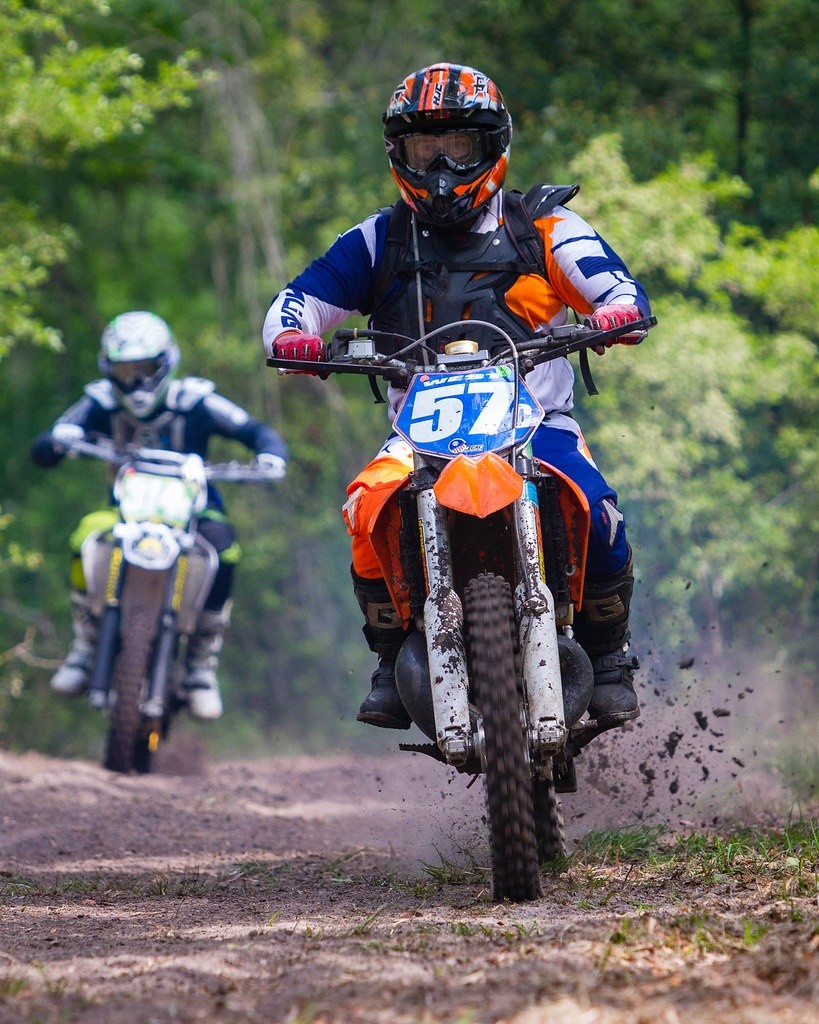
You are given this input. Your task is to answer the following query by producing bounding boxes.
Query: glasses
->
[394,127,490,181]
[103,352,169,391]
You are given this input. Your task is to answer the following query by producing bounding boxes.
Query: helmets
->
[100,312,179,419]
[383,63,513,227]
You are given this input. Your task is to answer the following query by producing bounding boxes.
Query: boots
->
[572,541,641,722]
[349,562,413,730]
[186,612,231,719]
[50,591,102,696]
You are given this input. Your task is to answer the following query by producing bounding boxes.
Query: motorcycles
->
[55,434,285,778]
[265,317,660,903]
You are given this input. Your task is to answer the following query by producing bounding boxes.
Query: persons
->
[29,311,286,723]
[261,62,651,729]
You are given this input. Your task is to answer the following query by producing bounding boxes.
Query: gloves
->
[273,333,326,376]
[257,452,284,482]
[585,303,648,356]
[55,423,83,451]
